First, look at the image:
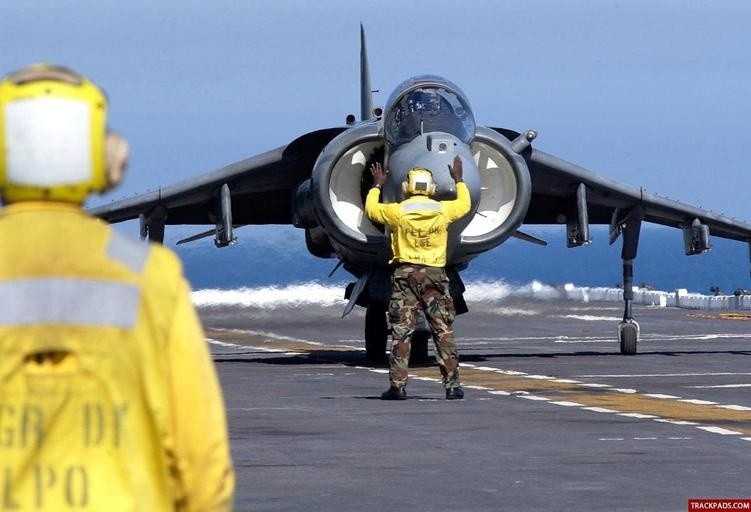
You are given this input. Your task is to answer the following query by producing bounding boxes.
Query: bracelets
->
[371,184,382,189]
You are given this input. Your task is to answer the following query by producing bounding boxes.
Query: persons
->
[0,65,236,512]
[365,154,472,400]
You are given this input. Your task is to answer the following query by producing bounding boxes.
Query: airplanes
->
[85,20,751,369]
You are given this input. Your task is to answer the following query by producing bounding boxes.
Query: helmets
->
[0,64,107,203]
[407,167,433,196]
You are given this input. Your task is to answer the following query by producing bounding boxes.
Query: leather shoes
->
[381,386,406,400]
[446,388,464,399]
